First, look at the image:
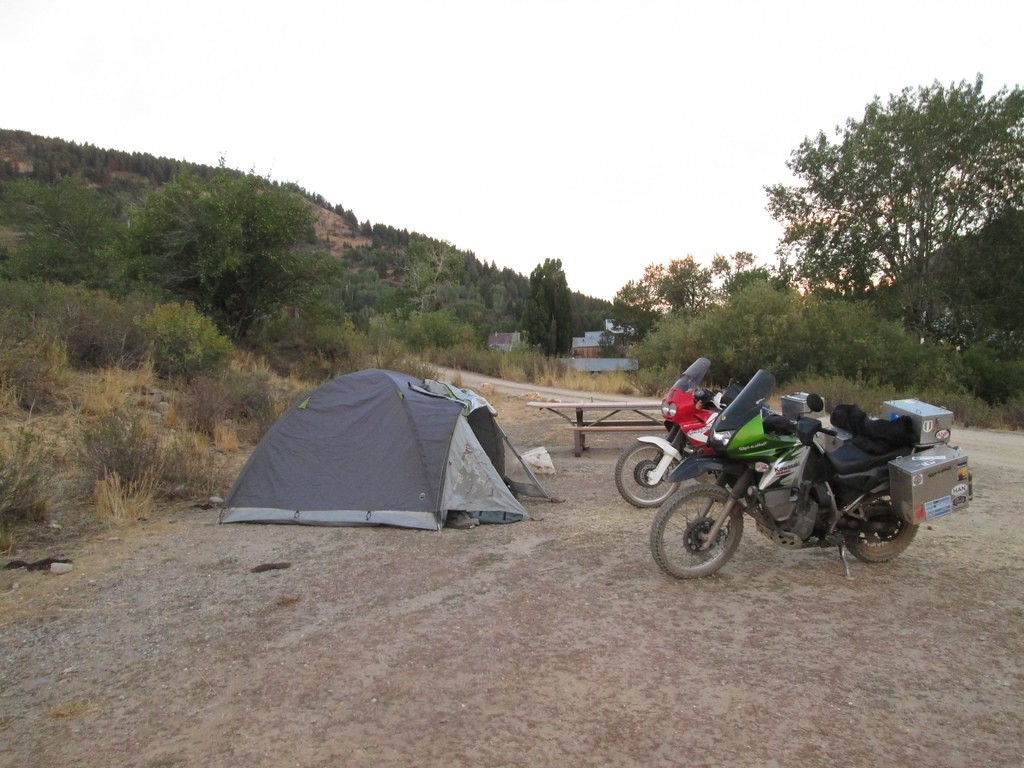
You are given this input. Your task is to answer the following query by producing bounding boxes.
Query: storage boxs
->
[883,397,954,445]
[779,390,825,419]
[888,445,970,524]
[825,416,879,452]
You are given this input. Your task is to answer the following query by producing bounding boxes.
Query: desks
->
[527,399,663,458]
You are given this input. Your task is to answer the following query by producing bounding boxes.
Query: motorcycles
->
[613,357,826,509]
[648,367,975,581]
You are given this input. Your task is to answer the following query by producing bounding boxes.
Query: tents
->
[216,369,550,532]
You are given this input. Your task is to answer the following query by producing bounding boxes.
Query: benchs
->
[571,419,666,426]
[563,425,668,432]
